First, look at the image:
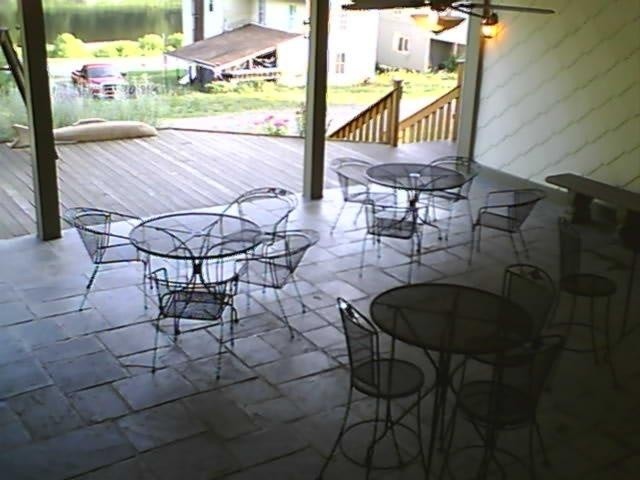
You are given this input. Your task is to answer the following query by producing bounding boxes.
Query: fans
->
[343,1,556,17]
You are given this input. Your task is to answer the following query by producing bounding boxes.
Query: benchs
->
[543,172,640,246]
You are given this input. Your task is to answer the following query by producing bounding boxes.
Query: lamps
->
[409,9,501,41]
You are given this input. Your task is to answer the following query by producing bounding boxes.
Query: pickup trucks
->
[70,63,128,100]
